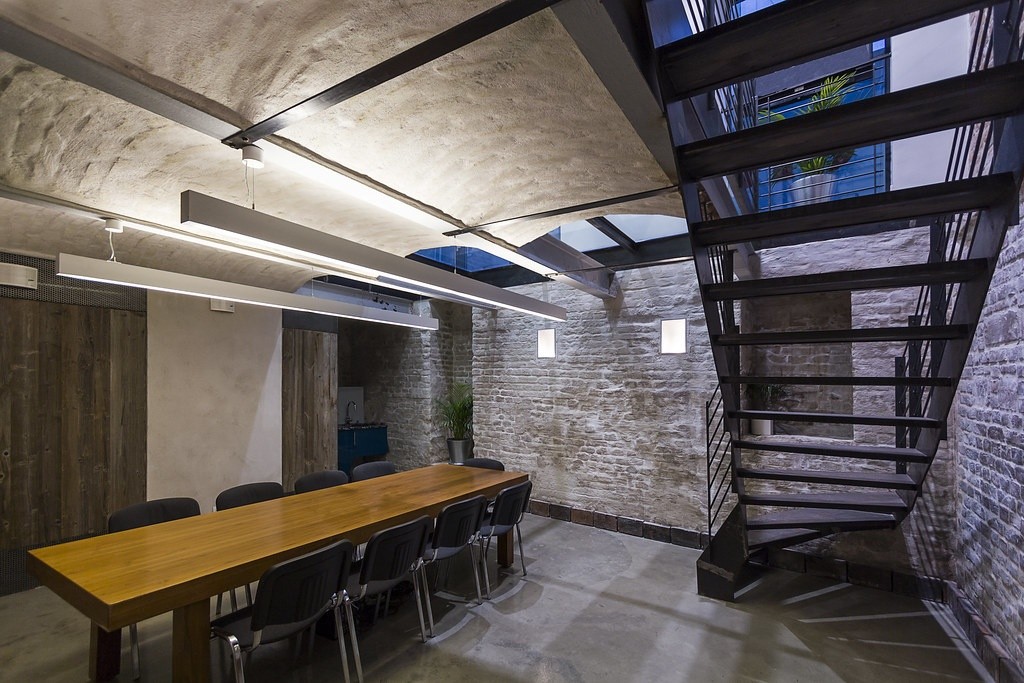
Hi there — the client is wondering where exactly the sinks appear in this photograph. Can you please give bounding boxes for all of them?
[338,427,350,430]
[350,426,377,430]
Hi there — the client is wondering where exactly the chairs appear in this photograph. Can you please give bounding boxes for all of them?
[209,539,356,682]
[309,514,427,683]
[444,480,532,598]
[108,497,201,680]
[352,460,396,483]
[383,494,488,637]
[463,458,505,560]
[214,481,285,617]
[295,470,349,495]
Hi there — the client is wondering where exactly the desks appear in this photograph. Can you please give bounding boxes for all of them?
[26,463,529,683]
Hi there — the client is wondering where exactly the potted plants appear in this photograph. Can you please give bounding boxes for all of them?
[744,366,805,435]
[758,67,873,205]
[434,382,473,463]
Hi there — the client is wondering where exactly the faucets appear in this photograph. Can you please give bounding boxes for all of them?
[344,400,357,424]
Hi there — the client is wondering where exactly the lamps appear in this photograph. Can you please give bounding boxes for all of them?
[54,218,437,331]
[179,143,566,323]
